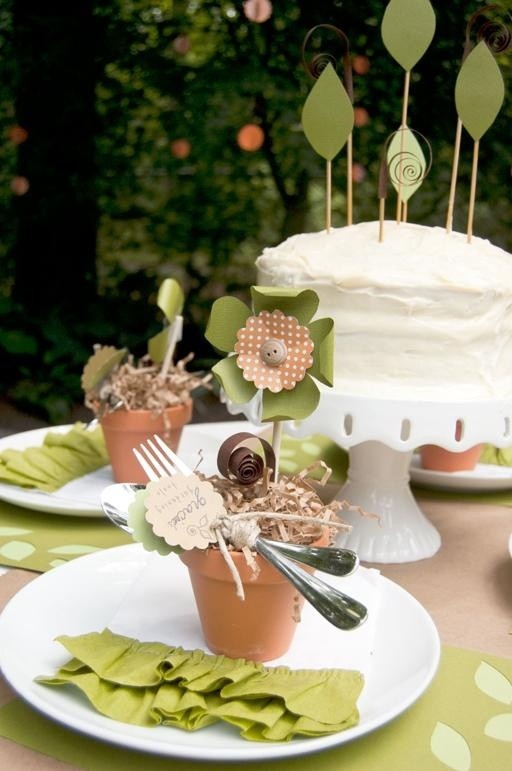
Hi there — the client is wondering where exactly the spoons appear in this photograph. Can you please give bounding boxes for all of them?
[101,483,360,577]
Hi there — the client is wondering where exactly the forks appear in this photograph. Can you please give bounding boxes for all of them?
[132,435,369,632]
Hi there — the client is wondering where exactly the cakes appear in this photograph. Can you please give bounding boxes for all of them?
[254,219,512,401]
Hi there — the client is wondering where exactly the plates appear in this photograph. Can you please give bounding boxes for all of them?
[408,455,512,494]
[1,423,117,518]
[0,541,441,764]
